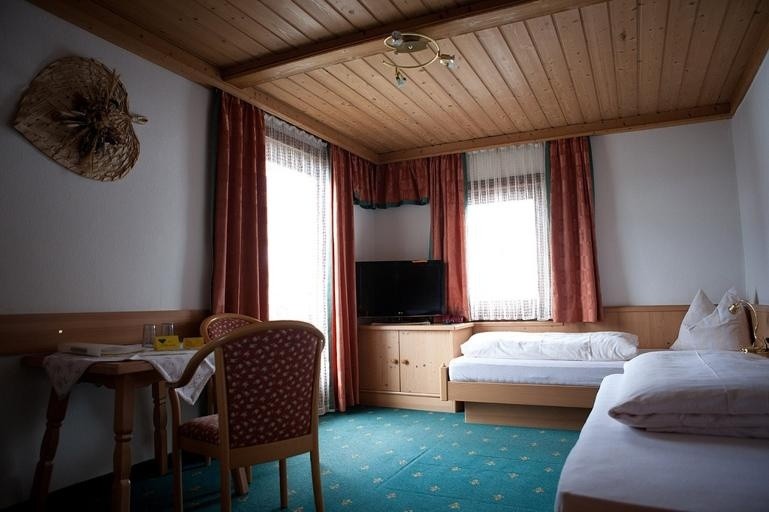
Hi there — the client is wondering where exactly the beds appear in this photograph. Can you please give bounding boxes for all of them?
[441,331,754,428]
[552,375,769,512]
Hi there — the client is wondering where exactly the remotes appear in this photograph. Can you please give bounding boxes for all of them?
[445,320,451,324]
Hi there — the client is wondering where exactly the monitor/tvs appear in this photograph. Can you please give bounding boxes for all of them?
[355,260,443,323]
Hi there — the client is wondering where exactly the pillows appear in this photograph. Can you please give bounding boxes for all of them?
[689,286,752,351]
[670,288,716,350]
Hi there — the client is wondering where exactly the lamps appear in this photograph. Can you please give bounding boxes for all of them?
[728,298,765,352]
[382,30,455,87]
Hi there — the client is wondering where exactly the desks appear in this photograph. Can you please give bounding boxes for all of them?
[30,348,248,512]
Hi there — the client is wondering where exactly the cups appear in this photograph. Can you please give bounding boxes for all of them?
[162,323,174,336]
[142,324,157,348]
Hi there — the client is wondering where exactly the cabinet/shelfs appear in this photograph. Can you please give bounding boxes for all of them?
[355,322,474,413]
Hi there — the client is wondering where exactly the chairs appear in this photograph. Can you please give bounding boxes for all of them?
[163,321,325,512]
[201,313,265,483]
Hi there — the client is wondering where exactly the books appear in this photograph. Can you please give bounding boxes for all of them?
[57,341,143,355]
